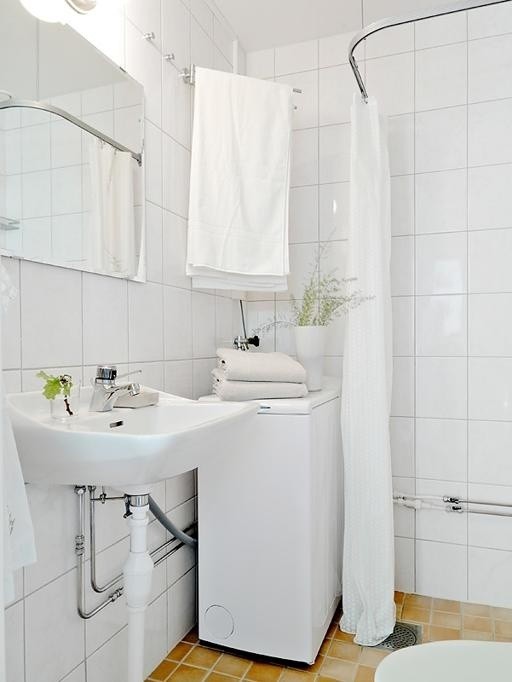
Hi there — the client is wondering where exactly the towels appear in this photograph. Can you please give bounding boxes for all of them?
[209,370,308,401]
[217,347,308,383]
[185,61,297,293]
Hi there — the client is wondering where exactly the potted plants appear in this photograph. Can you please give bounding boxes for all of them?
[251,230,376,393]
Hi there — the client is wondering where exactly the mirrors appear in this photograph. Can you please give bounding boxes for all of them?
[1,0,152,287]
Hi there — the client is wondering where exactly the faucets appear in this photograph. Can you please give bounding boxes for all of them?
[87,364,140,413]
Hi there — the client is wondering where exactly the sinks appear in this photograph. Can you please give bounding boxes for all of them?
[4,384,262,489]
